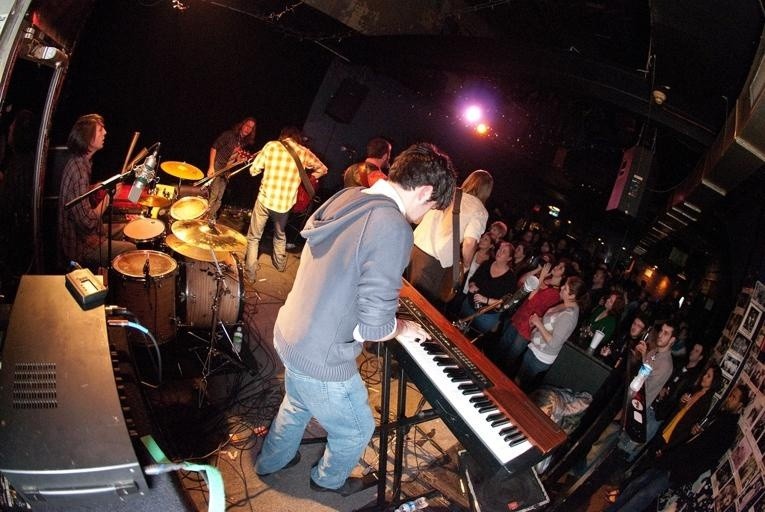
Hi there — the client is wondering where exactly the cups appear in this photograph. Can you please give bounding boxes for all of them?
[699,415,709,428]
[685,392,691,401]
[642,327,657,345]
[589,329,605,349]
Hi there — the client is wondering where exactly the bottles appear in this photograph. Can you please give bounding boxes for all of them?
[629,354,655,393]
[474,290,482,311]
[232,325,247,354]
[394,497,430,512]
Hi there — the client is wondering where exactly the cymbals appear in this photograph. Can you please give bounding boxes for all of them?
[161,161,204,180]
[171,196,208,221]
[166,232,231,262]
[138,196,171,207]
[171,218,248,252]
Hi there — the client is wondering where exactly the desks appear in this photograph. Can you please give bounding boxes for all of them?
[543,333,616,395]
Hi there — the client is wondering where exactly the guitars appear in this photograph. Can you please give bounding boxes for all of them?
[440,259,464,302]
[239,150,318,214]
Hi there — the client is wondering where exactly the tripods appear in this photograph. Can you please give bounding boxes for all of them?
[267,137,310,244]
[183,281,258,407]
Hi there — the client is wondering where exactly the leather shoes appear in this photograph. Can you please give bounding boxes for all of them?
[309,477,364,494]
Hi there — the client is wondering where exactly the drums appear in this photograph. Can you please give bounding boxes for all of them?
[124,218,165,249]
[182,253,244,331]
[113,250,178,343]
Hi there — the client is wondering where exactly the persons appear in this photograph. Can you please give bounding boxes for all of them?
[407,170,493,320]
[207,117,257,223]
[450,207,750,511]
[342,138,392,189]
[0,109,38,287]
[244,126,328,284]
[254,143,458,495]
[58,114,137,270]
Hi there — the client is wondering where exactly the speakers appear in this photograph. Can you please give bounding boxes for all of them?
[457,447,551,512]
[323,78,371,124]
[538,333,615,401]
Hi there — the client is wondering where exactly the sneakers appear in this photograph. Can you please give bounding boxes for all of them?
[255,450,302,477]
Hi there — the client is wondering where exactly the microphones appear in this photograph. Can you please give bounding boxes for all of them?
[498,275,541,312]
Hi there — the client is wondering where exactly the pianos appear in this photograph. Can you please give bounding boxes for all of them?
[382,275,567,484]
